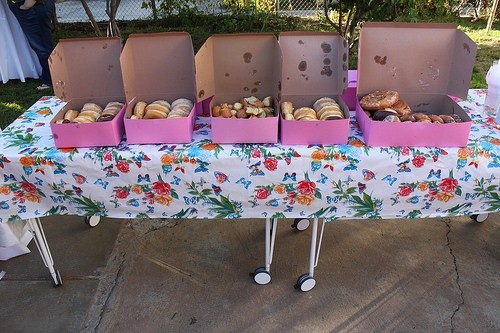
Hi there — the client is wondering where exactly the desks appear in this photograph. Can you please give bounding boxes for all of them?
[0,89,500,292]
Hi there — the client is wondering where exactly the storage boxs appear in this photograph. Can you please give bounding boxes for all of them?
[354,22,478,147]
[47,38,127,148]
[121,31,195,144]
[341,69,360,109]
[280,32,350,144]
[195,33,282,144]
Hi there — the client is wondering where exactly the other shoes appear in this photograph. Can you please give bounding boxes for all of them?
[36,85,50,90]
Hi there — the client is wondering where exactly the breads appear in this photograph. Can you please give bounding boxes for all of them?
[55,101,125,123]
[359,90,463,124]
[212,95,274,119]
[280,97,344,120]
[129,98,193,119]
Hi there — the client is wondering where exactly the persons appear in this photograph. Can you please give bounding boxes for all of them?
[7,0,56,90]
